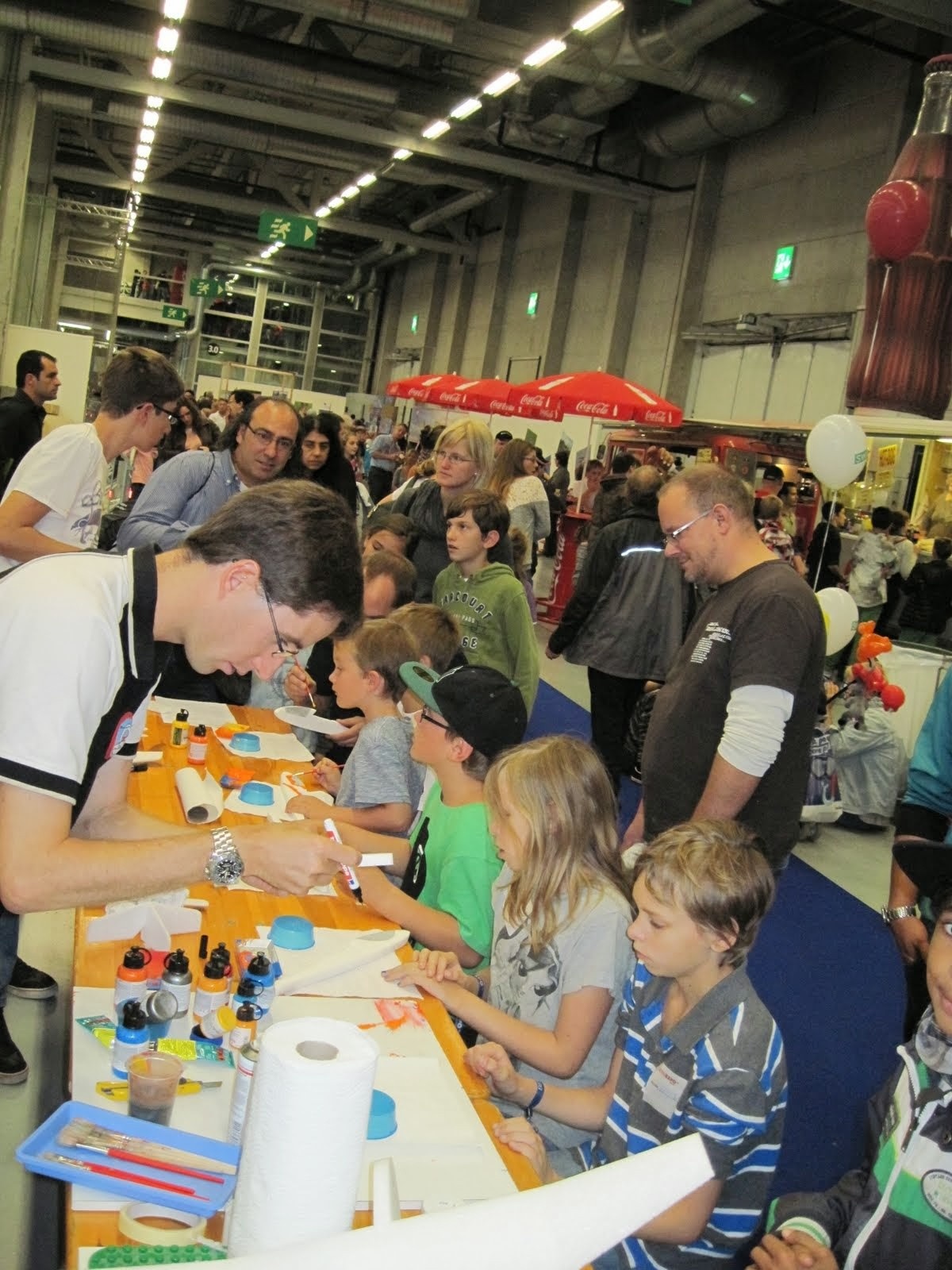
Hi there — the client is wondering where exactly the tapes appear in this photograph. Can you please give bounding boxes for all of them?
[118,1201,207,1247]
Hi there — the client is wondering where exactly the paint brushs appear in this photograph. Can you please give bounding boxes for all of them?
[294,655,316,707]
[37,1150,210,1200]
[290,765,345,777]
[57,1118,237,1183]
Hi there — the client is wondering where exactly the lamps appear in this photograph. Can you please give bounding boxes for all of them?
[261,0,626,259]
[117,0,187,247]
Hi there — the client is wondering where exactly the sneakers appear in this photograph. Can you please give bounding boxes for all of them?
[3,954,58,999]
[0,1011,29,1084]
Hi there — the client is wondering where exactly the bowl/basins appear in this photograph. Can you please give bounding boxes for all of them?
[266,916,316,950]
[238,783,275,805]
[117,997,172,1041]
[229,734,261,752]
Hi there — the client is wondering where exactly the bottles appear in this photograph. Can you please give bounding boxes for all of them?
[113,1000,151,1079]
[243,951,277,1014]
[229,1000,264,1048]
[367,1088,400,1139]
[134,991,178,1023]
[194,1006,237,1039]
[188,724,208,765]
[113,945,151,1012]
[228,1032,264,1146]
[193,942,234,1020]
[169,708,192,748]
[231,976,264,1009]
[159,948,192,1019]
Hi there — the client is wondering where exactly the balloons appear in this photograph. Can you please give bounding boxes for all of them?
[865,180,931,266]
[816,587,859,657]
[805,414,868,490]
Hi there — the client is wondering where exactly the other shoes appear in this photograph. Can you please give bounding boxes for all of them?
[536,549,544,555]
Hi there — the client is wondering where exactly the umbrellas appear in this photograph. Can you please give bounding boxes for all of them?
[422,377,564,423]
[386,373,510,416]
[505,370,682,428]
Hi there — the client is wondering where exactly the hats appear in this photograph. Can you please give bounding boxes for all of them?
[398,661,527,762]
[763,464,784,480]
[890,826,952,897]
[495,430,512,441]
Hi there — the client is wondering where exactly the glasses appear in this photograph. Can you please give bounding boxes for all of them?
[432,449,477,465]
[522,455,536,463]
[136,404,181,425]
[231,558,300,659]
[417,704,454,735]
[246,422,296,452]
[662,509,714,548]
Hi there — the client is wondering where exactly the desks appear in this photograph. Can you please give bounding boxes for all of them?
[67,701,592,1270]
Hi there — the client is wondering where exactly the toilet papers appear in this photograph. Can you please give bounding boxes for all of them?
[227,1014,380,1257]
[175,765,224,824]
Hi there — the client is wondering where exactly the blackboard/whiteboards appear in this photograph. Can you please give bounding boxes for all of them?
[408,407,468,444]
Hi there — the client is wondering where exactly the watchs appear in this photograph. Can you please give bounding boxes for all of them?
[879,904,915,927]
[203,827,244,888]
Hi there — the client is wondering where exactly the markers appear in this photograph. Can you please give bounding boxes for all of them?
[131,764,147,771]
[324,818,363,905]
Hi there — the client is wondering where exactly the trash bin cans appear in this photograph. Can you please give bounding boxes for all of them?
[875,640,952,800]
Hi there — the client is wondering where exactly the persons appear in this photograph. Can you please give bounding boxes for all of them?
[0,346,952,1270]
[466,816,788,1270]
[618,464,828,879]
[430,484,541,727]
[744,885,952,1270]
[0,481,364,919]
[0,350,62,503]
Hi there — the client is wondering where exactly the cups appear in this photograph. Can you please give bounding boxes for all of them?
[126,1052,184,1128]
[190,1027,223,1046]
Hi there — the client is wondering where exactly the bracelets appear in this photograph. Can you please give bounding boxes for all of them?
[473,973,484,999]
[518,1080,545,1120]
